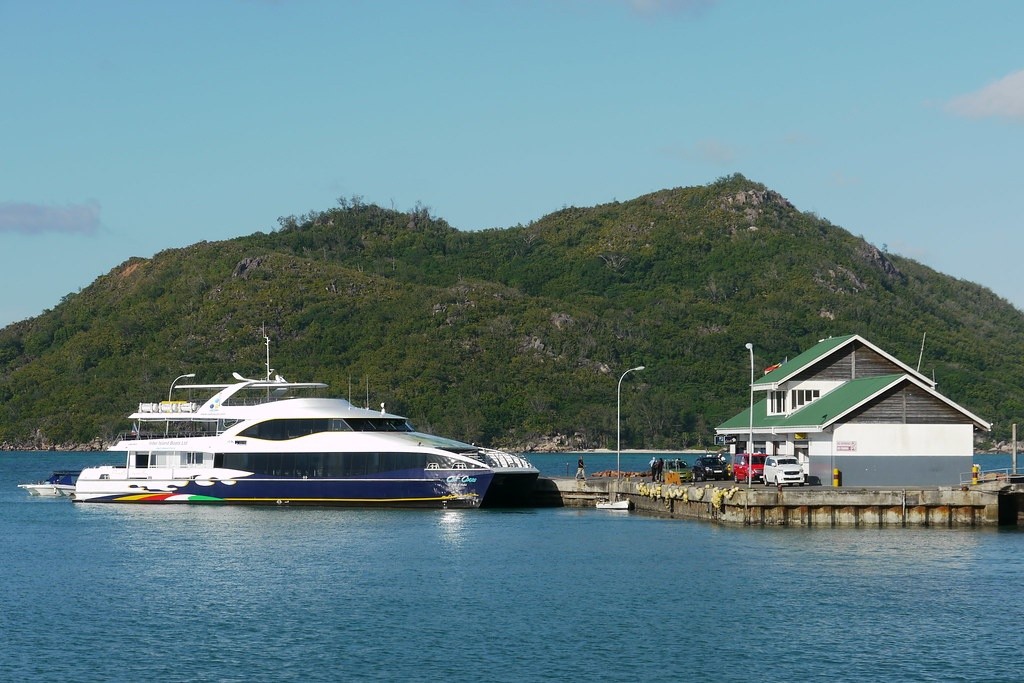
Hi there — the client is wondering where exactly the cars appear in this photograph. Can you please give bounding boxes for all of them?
[661,459,693,483]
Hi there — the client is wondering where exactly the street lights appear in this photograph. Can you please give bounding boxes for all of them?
[744,341,755,489]
[163,372,196,437]
[617,364,646,498]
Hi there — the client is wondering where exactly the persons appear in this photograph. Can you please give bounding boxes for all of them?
[575,456,587,480]
[649,457,664,482]
[719,454,725,461]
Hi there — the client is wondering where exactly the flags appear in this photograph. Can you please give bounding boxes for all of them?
[131,425,136,435]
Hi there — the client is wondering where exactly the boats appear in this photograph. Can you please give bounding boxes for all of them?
[70,333,540,508]
[16,470,82,498]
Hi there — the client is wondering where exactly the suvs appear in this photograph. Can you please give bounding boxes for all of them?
[693,457,729,482]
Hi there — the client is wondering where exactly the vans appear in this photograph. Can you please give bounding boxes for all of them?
[763,455,805,487]
[733,454,768,484]
